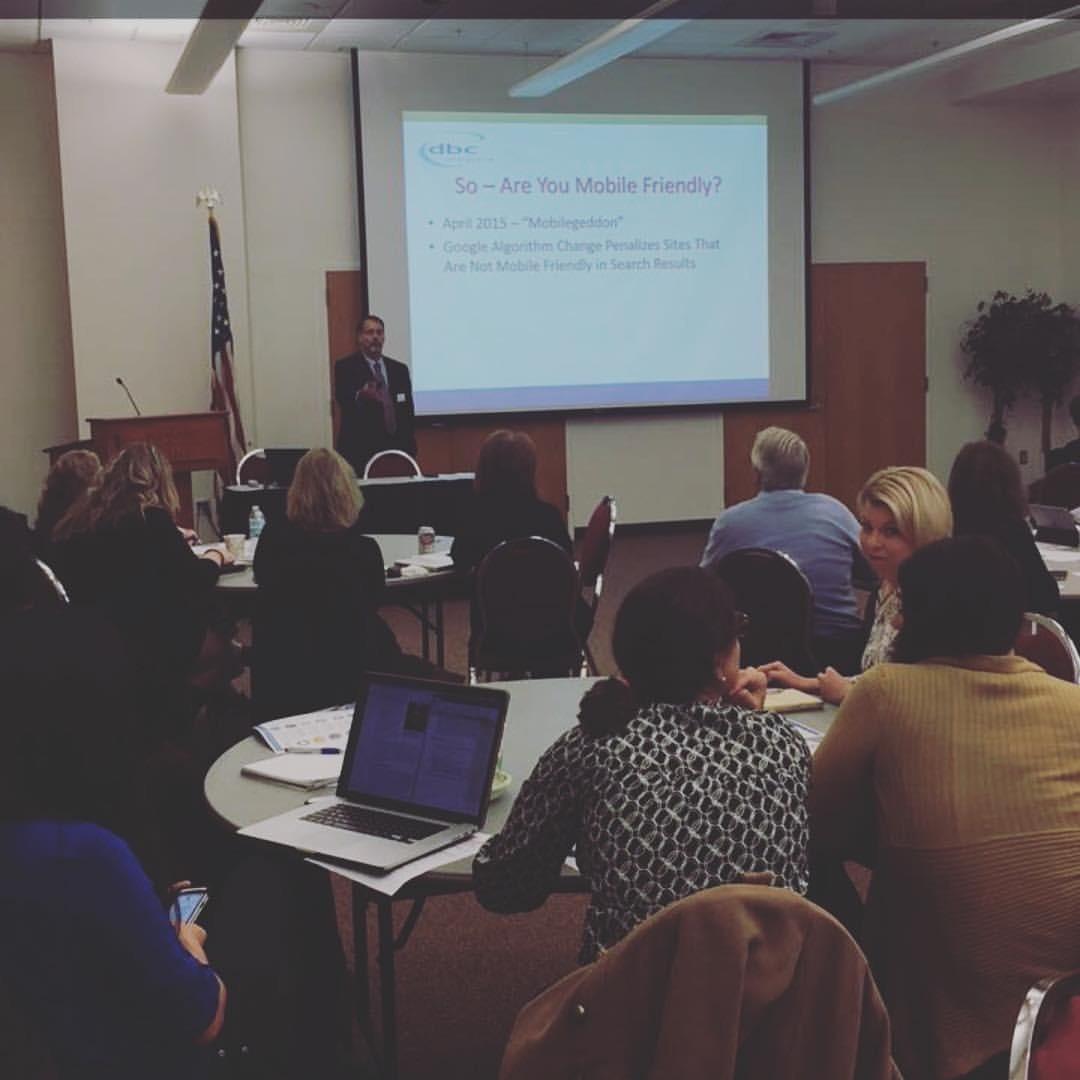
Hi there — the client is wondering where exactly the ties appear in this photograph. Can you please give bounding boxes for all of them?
[373,363,397,437]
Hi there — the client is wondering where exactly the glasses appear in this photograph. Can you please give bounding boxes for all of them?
[727,610,752,642]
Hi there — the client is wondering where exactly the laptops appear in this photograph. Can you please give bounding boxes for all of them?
[236,670,512,872]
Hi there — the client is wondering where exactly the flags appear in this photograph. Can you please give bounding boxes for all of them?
[207,217,249,540]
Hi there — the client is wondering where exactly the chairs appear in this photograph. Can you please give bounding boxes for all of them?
[1009,971,1080,1079]
[24,492,620,677]
[494,880,894,1080]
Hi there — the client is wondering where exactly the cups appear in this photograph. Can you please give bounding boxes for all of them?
[224,534,246,561]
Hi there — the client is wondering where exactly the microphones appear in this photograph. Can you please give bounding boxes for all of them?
[116,376,141,417]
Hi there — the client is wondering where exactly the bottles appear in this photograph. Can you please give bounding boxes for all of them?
[249,505,265,552]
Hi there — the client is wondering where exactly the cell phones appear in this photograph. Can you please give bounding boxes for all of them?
[169,886,212,927]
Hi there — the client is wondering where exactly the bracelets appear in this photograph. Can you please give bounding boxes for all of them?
[203,547,225,571]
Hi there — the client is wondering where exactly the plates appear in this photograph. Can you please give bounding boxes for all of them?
[491,770,512,800]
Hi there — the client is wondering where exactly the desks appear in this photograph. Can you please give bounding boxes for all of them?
[221,470,486,543]
[205,678,843,1080]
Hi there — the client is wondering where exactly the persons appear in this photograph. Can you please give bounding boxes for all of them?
[754,465,957,709]
[331,315,417,479]
[51,442,239,766]
[947,442,1063,624]
[1044,396,1080,472]
[696,424,879,681]
[805,530,1080,1080]
[450,430,590,678]
[253,445,409,720]
[27,449,107,578]
[1,673,230,1079]
[471,566,816,969]
